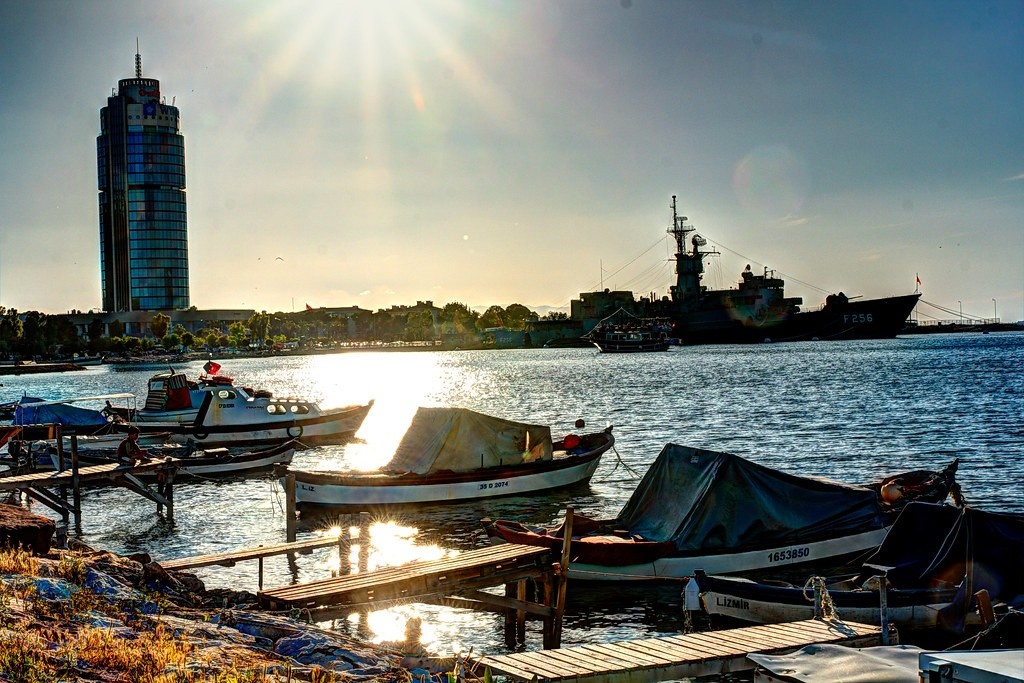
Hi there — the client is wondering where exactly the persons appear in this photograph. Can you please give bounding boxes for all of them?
[117,426,152,465]
[599,321,673,341]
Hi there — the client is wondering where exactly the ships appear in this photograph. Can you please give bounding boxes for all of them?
[481,194,923,349]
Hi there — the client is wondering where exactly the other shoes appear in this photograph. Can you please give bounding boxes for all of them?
[141,457,153,464]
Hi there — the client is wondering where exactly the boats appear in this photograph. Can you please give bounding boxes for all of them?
[678,474,1024,632]
[273,407,616,505]
[45,436,301,478]
[578,306,682,352]
[479,437,969,583]
[0,349,378,470]
[744,588,1024,683]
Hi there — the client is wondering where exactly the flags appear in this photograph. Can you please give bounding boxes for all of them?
[209,360,221,374]
[916,277,921,284]
[306,304,312,310]
